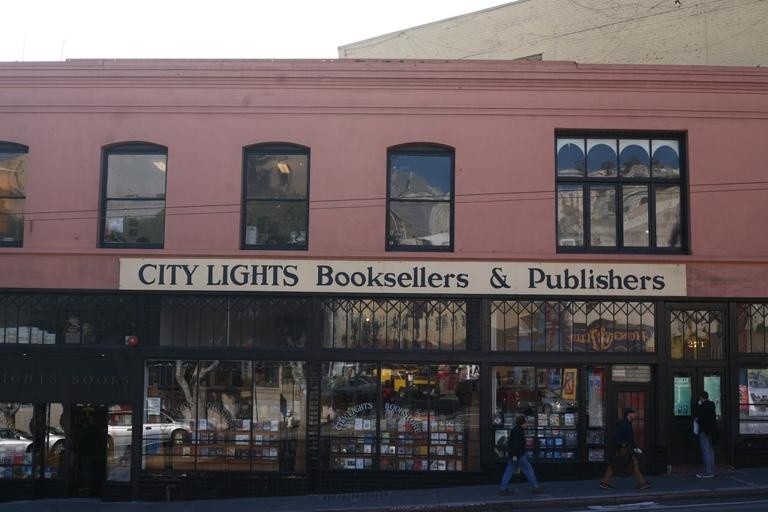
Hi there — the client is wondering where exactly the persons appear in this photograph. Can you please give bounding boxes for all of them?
[495,414,544,496]
[597,407,655,492]
[691,391,720,480]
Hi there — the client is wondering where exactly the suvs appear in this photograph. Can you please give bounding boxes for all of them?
[497,384,536,411]
[107,408,192,451]
[371,365,440,400]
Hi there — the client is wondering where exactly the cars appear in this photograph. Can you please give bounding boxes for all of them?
[532,388,577,414]
[322,374,394,411]
[44,426,67,457]
[454,375,480,405]
[0,427,34,466]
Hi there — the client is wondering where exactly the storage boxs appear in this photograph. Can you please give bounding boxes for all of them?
[144,425,304,471]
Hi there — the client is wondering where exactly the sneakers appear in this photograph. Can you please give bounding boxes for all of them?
[640,481,653,490]
[600,480,616,490]
[696,472,714,479]
